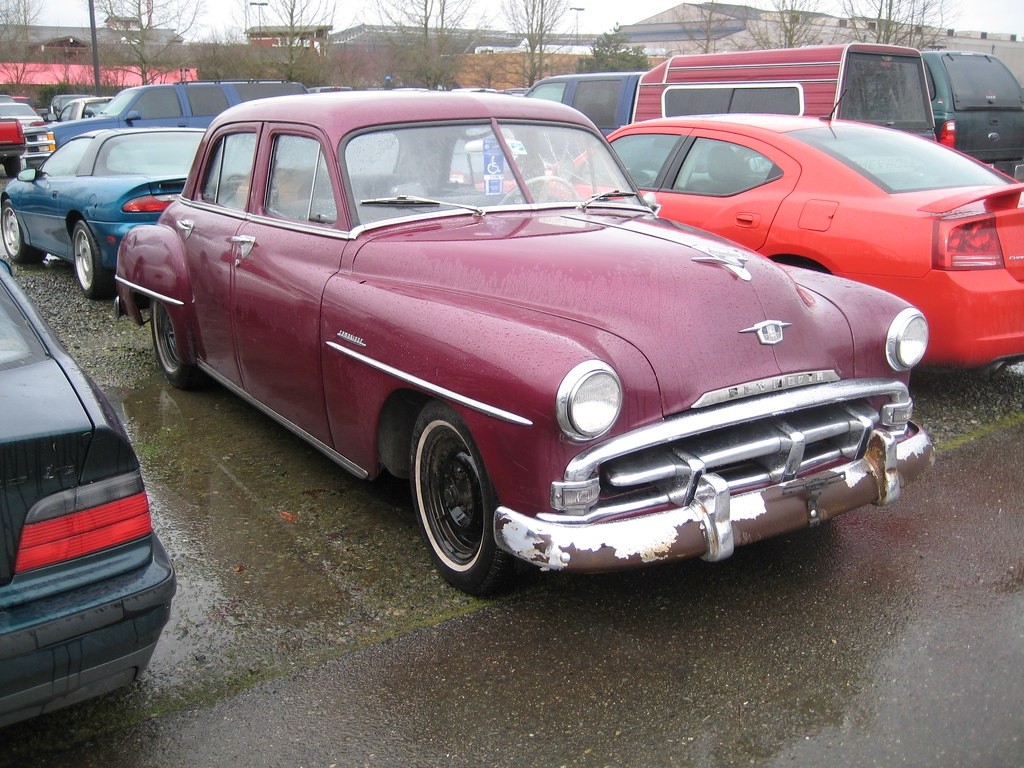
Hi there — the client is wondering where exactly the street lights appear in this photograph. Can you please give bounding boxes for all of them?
[569,7,585,45]
[249,2,269,48]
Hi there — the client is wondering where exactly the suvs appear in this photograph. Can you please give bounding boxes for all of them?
[919,51,1023,189]
[18,78,312,181]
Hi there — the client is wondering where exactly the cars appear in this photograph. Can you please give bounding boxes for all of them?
[0,103,45,136]
[107,88,933,603]
[12,97,37,114]
[0,126,245,298]
[0,264,179,731]
[0,95,16,103]
[51,96,116,125]
[43,94,97,126]
[500,110,1024,378]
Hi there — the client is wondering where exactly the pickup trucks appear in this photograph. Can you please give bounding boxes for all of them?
[0,118,26,179]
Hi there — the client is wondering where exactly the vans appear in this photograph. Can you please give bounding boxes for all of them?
[509,42,939,165]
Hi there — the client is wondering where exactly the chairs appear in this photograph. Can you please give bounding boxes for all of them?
[701,144,751,193]
